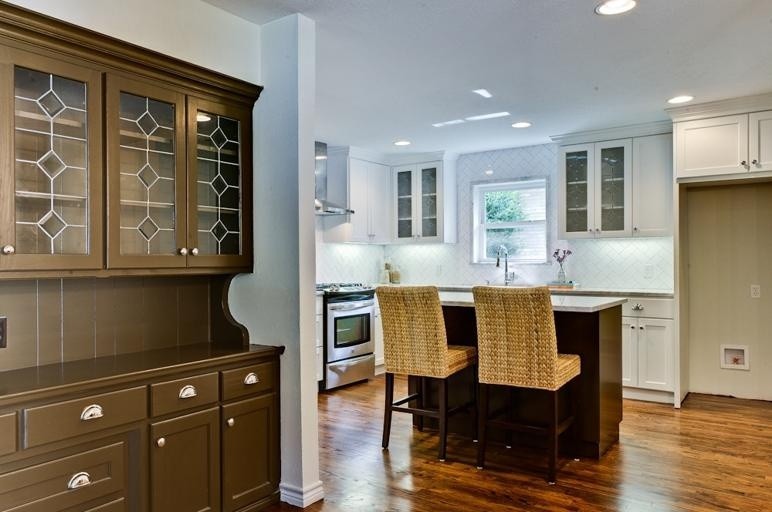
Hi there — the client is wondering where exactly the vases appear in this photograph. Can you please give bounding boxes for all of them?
[558,263,565,281]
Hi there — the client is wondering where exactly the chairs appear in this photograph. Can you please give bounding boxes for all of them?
[375,285,478,462]
[472,285,585,486]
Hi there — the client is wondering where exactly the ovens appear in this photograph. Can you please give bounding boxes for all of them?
[323,294,376,392]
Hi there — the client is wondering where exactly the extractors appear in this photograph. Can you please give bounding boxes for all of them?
[315,141,355,218]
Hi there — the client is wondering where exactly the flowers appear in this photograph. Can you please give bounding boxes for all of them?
[552,248,572,266]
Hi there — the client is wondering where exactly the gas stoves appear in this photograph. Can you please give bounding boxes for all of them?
[315,281,377,297]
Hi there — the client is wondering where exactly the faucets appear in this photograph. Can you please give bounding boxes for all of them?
[495,246,515,286]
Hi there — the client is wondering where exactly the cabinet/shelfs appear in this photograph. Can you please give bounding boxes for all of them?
[0,384,148,512]
[104,72,253,270]
[556,138,632,240]
[148,370,220,512]
[622,296,675,393]
[632,132,674,239]
[316,296,324,382]
[391,150,458,245]
[0,43,104,272]
[673,110,772,179]
[220,359,281,512]
[323,144,391,245]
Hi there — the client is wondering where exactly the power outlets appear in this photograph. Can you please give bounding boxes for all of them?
[750,284,760,297]
